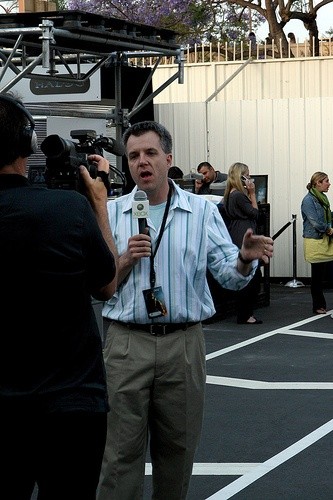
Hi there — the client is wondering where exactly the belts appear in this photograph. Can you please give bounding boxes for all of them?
[116,320,196,337]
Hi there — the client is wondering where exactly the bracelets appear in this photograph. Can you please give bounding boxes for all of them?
[238,251,252,266]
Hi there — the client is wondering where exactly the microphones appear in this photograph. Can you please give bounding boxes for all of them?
[101,136,125,156]
[133,190,149,236]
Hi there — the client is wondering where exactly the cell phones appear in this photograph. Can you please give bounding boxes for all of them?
[240,176,246,186]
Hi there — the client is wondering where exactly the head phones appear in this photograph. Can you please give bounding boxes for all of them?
[0,94,39,158]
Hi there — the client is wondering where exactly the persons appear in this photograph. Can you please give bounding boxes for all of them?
[167,167,184,190]
[194,162,228,198]
[0,90,118,500]
[98,121,275,500]
[301,172,333,314]
[223,163,264,324]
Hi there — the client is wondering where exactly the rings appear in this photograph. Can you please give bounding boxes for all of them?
[264,249,266,254]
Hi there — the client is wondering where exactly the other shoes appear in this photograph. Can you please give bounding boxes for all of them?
[240,316,262,324]
[315,307,326,314]
